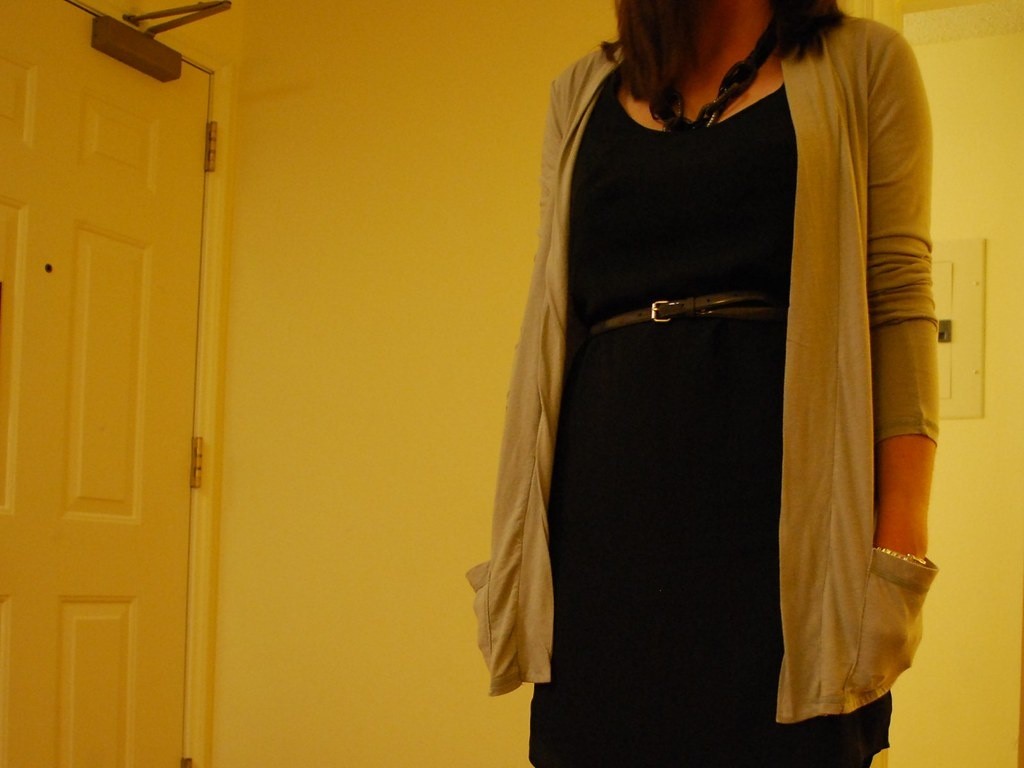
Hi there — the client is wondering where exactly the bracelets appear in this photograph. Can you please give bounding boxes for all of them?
[877,547,926,566]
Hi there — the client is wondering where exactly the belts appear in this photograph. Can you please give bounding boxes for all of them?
[586,289,784,336]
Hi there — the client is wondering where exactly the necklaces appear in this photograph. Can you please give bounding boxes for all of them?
[649,16,779,133]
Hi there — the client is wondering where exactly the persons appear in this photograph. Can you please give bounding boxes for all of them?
[466,0,939,768]
